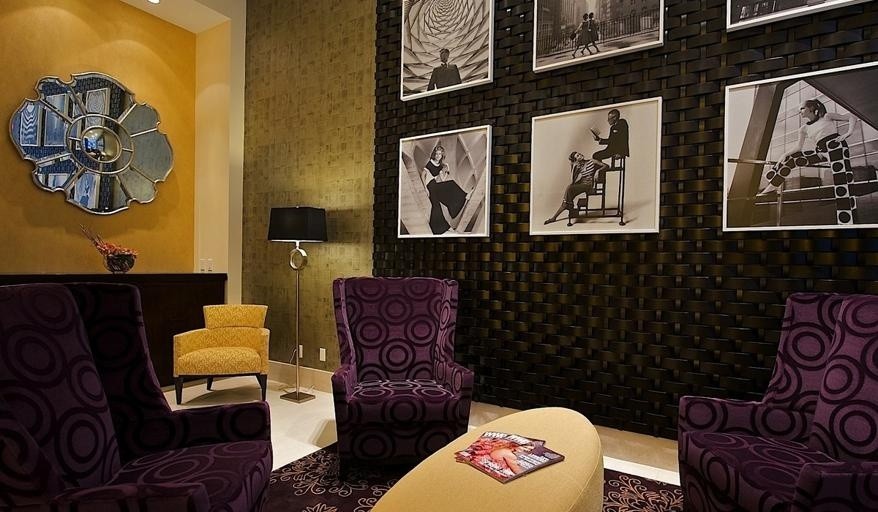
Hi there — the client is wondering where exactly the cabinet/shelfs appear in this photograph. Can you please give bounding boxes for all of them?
[2,273,228,389]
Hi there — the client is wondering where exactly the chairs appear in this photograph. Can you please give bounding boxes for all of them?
[673,291,877,510]
[0,281,275,511]
[68,284,274,459]
[330,274,475,477]
[171,304,271,407]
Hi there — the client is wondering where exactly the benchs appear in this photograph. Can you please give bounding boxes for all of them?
[366,400,606,512]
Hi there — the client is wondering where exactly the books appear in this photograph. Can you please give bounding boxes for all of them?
[455,434,565,484]
[455,429,546,465]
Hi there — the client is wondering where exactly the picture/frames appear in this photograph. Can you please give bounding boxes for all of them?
[73,170,103,211]
[400,1,496,104]
[20,93,44,149]
[720,62,878,232]
[43,94,71,146]
[532,1,665,76]
[725,0,876,36]
[47,172,72,187]
[528,97,663,235]
[85,88,113,132]
[397,125,493,239]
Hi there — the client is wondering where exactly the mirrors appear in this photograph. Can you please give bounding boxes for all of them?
[8,71,174,216]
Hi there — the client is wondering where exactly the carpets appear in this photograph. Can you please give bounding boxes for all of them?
[259,440,688,512]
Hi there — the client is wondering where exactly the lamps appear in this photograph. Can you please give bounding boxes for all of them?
[267,205,328,405]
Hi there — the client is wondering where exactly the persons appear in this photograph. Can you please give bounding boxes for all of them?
[571,14,588,55]
[477,436,534,474]
[582,14,601,56]
[758,99,860,227]
[426,49,462,94]
[589,108,631,176]
[420,145,469,235]
[545,151,609,224]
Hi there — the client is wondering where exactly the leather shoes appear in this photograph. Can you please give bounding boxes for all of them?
[756,191,777,199]
